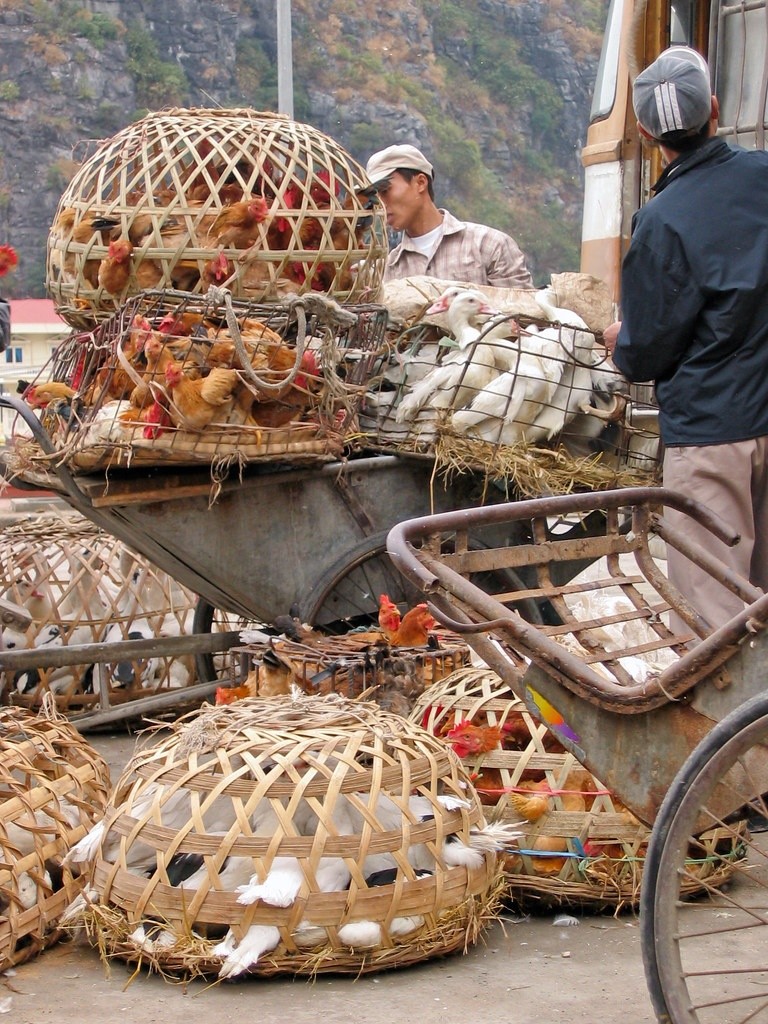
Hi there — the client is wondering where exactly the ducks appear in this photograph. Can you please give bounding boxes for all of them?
[363,287,620,458]
[0,771,528,980]
[2,536,271,699]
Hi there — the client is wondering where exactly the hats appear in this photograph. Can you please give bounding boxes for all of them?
[632,45,712,143]
[344,144,434,203]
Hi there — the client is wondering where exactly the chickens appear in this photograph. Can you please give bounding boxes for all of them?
[217,594,649,891]
[15,148,384,449]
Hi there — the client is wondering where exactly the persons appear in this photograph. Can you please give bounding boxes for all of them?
[362,142,535,291]
[603,45,767,653]
